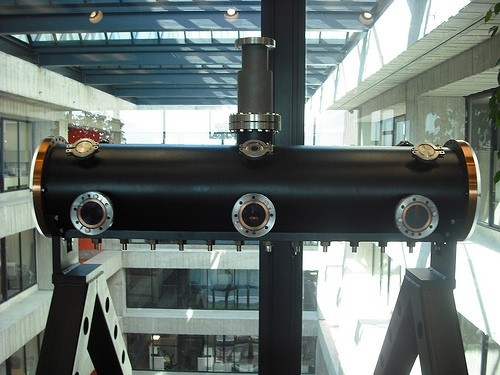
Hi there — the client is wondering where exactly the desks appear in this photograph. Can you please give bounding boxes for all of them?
[4,174,28,191]
